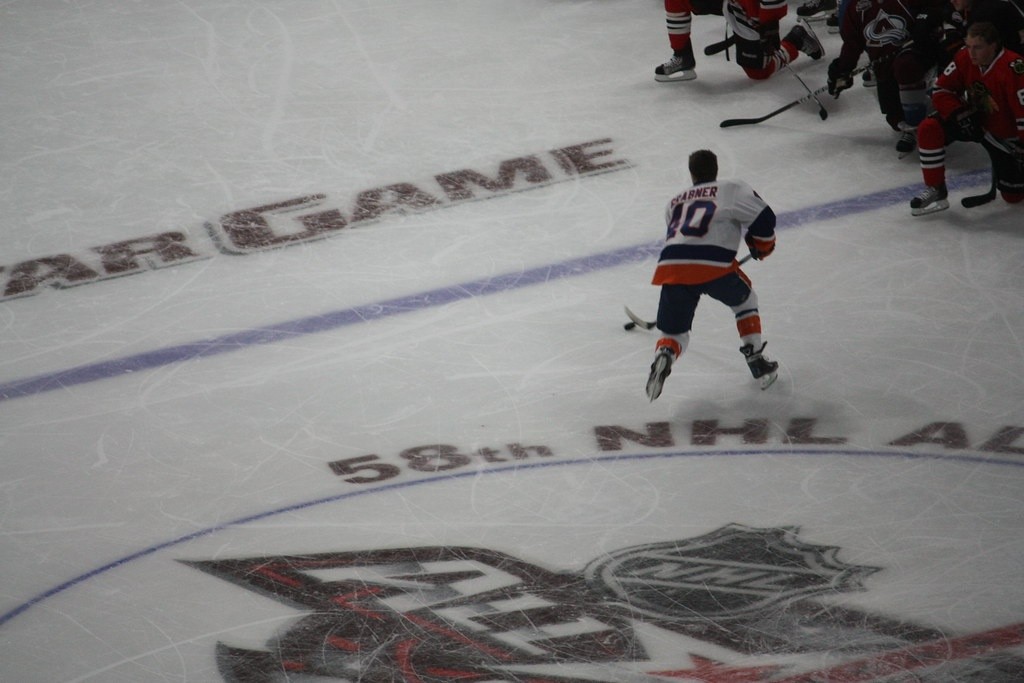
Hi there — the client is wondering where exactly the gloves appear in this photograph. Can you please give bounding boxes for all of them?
[827,59,853,99]
[995,151,1024,194]
[952,106,989,144]
[747,244,774,260]
[911,11,944,50]
[759,20,780,56]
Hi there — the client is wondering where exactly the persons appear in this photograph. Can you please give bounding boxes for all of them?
[654,0,1024,161]
[909,22,1024,216]
[646,150,778,403]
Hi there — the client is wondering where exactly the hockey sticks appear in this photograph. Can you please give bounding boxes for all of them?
[720,49,900,128]
[624,253,752,331]
[732,0,828,121]
[704,34,735,56]
[961,160,998,209]
[725,22,730,61]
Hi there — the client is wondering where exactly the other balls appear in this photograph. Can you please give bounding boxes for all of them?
[624,321,635,331]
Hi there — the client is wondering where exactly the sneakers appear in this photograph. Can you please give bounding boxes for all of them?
[782,19,825,60]
[655,38,697,82]
[909,177,949,216]
[896,121,919,159]
[862,68,877,87]
[796,0,838,22]
[740,341,779,390]
[646,352,672,402]
[826,12,841,35]
